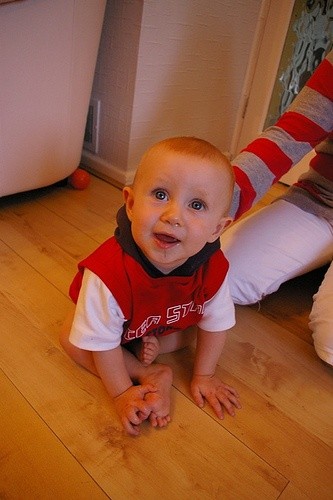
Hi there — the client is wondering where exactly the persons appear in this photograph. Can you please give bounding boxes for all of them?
[220,47,333,365]
[59,136,240,436]
